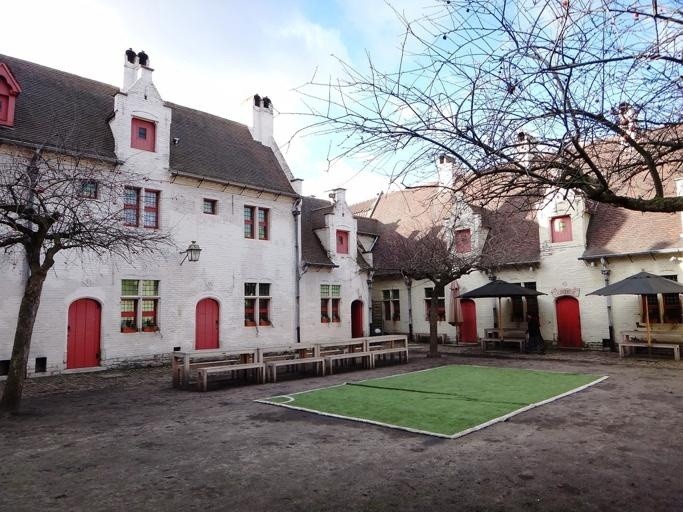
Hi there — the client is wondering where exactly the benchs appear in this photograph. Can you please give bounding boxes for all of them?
[172,328,408,391]
[415,331,447,345]
[618,330,683,360]
[480,327,530,354]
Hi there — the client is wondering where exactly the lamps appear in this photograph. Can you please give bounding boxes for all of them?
[179,240,202,265]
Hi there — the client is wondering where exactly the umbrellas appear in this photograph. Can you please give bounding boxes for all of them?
[452,276,547,337]
[583,268,683,347]
